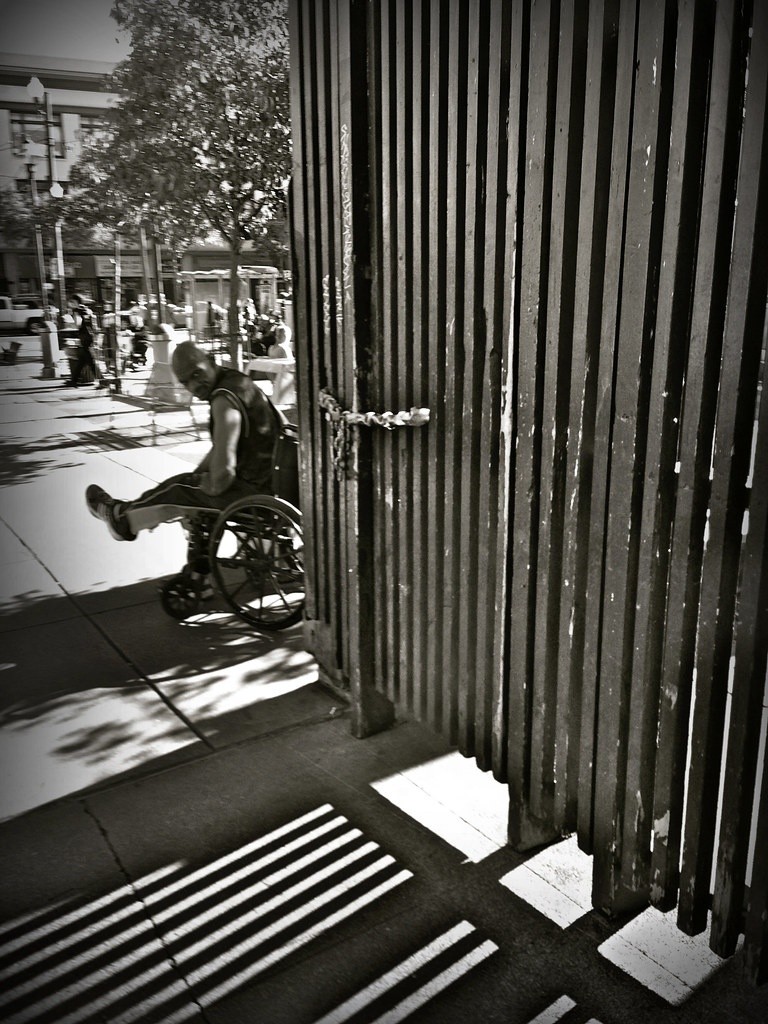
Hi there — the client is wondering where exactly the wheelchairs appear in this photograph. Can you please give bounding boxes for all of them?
[156,419,307,631]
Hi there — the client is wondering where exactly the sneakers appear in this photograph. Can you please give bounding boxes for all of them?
[160,570,215,600]
[86,484,137,541]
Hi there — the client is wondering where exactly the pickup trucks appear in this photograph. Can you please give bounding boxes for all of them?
[0,296,47,337]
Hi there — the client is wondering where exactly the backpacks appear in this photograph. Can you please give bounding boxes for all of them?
[78,314,94,347]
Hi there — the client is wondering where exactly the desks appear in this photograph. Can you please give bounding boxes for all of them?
[245,359,295,407]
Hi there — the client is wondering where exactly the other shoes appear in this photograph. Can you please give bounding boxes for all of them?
[66,381,79,389]
[95,381,109,389]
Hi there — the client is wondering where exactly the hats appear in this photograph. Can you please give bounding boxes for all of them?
[272,310,282,318]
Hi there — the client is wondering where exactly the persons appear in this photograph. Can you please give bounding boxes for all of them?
[43,297,186,329]
[63,292,109,390]
[118,316,149,360]
[236,297,291,356]
[85,341,294,602]
[206,301,216,338]
[100,305,117,374]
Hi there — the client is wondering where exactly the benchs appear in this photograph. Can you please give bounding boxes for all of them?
[109,362,203,446]
